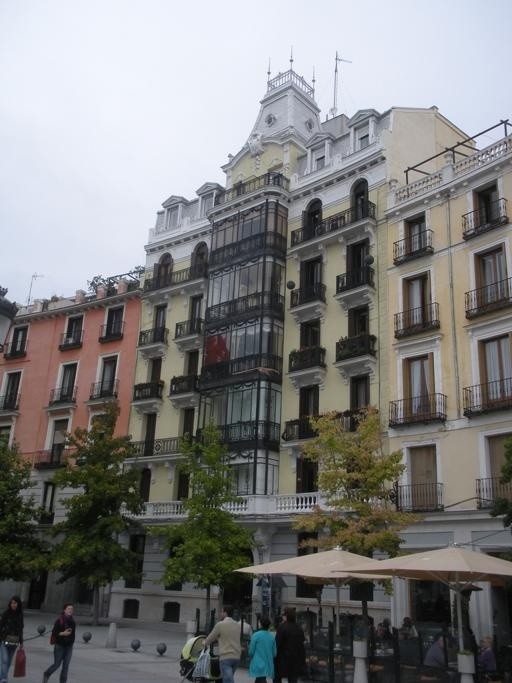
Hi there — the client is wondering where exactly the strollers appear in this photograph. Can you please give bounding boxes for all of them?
[179,636,221,682]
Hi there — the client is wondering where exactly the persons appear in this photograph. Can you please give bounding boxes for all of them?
[42,603,75,683]
[202,606,497,683]
[0,596,24,683]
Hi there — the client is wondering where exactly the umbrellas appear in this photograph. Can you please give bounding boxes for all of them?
[331,543,512,652]
[233,545,398,635]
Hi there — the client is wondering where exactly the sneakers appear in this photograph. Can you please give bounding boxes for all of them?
[43,672,49,683]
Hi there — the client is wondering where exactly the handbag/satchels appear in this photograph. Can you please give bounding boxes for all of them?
[192,644,212,679]
[51,617,63,644]
[13,647,26,677]
[3,634,19,648]
